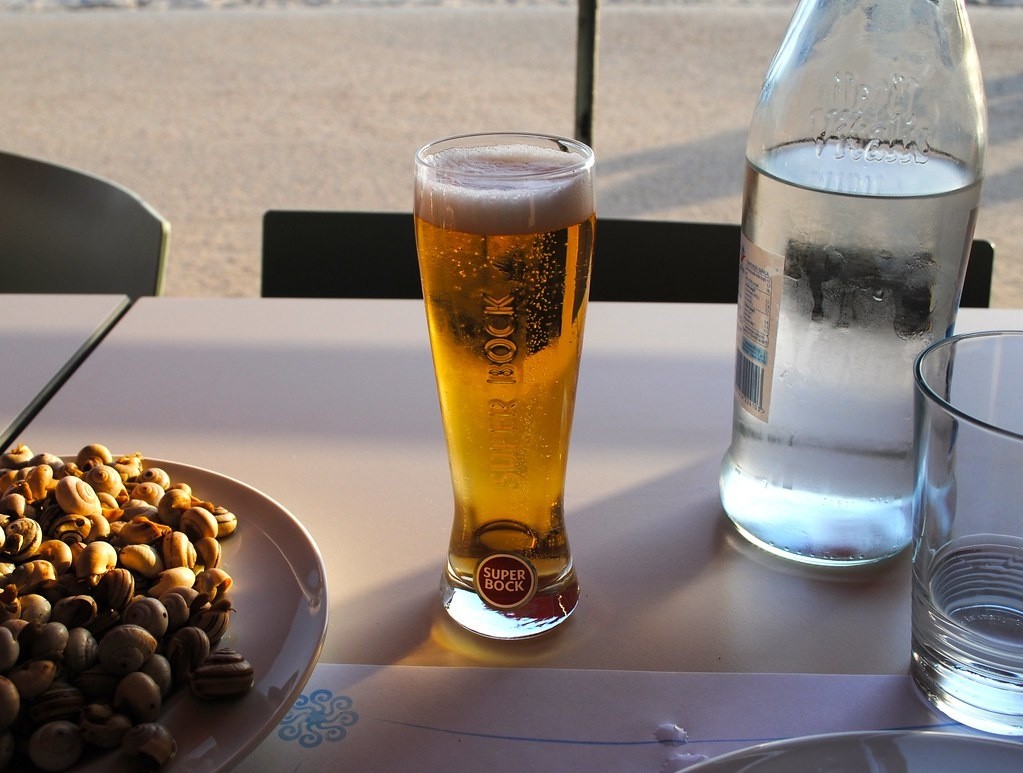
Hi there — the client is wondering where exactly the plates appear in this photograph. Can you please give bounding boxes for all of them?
[676,729,1023,773]
[55,455,330,773]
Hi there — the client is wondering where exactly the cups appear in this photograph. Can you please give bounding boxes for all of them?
[415,132,597,640]
[910,330,1023,738]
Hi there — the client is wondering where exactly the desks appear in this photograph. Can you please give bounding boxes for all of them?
[0,289,1023,773]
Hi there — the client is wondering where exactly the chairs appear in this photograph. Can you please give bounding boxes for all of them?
[0,151,170,296]
[259,209,995,308]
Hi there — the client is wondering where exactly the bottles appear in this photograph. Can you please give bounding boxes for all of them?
[719,0,988,567]
